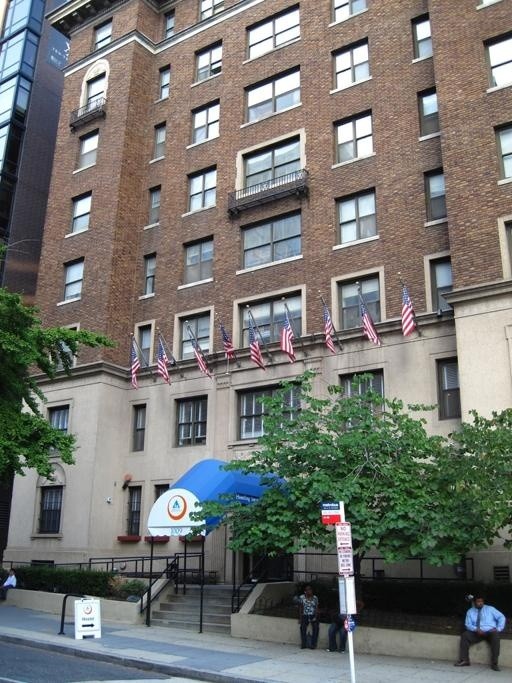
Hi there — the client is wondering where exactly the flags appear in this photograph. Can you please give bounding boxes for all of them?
[186,324,213,381]
[217,322,235,362]
[358,301,382,348]
[398,285,417,338]
[129,339,143,391]
[277,308,299,364]
[154,334,172,385]
[248,313,267,370]
[321,302,337,354]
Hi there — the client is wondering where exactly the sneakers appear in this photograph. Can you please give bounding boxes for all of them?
[326,647,345,654]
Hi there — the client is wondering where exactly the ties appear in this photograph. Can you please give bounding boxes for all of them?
[476,608,481,628]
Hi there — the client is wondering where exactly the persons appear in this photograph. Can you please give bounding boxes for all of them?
[0,567,8,587]
[454,592,508,672]
[296,582,323,651]
[327,597,365,652]
[0,567,17,600]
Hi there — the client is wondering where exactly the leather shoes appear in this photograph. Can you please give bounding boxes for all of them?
[454,659,470,666]
[490,663,500,671]
